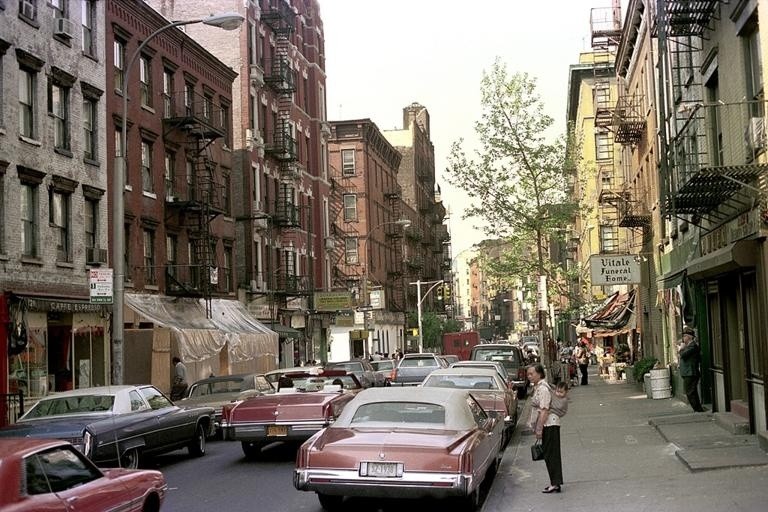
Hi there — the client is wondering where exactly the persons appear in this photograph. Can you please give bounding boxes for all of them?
[353,349,402,360]
[523,341,597,385]
[551,382,568,417]
[171,357,188,401]
[677,328,704,411]
[525,363,563,493]
[295,359,316,366]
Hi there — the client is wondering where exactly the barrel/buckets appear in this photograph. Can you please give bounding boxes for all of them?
[649,367,672,400]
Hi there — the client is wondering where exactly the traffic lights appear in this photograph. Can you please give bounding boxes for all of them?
[437,287,443,301]
[446,287,451,300]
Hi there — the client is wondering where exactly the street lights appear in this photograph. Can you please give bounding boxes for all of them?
[110,12,245,385]
[364,219,411,306]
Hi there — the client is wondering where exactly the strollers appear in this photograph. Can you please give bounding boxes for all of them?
[561,357,579,387]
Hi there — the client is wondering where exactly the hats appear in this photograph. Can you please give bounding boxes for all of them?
[683,329,696,338]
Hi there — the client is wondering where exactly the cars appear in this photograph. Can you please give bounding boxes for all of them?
[293,386,506,510]
[391,343,529,450]
[0,438,167,511]
[1,383,215,469]
[167,359,398,458]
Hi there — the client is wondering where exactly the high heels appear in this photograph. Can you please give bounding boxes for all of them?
[542,485,561,493]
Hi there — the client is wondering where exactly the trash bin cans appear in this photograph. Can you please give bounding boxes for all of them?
[650,369,672,399]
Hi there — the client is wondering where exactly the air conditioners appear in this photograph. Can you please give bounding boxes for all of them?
[54,18,76,39]
[742,117,764,155]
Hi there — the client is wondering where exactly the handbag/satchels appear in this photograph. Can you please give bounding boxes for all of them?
[531,439,544,461]
